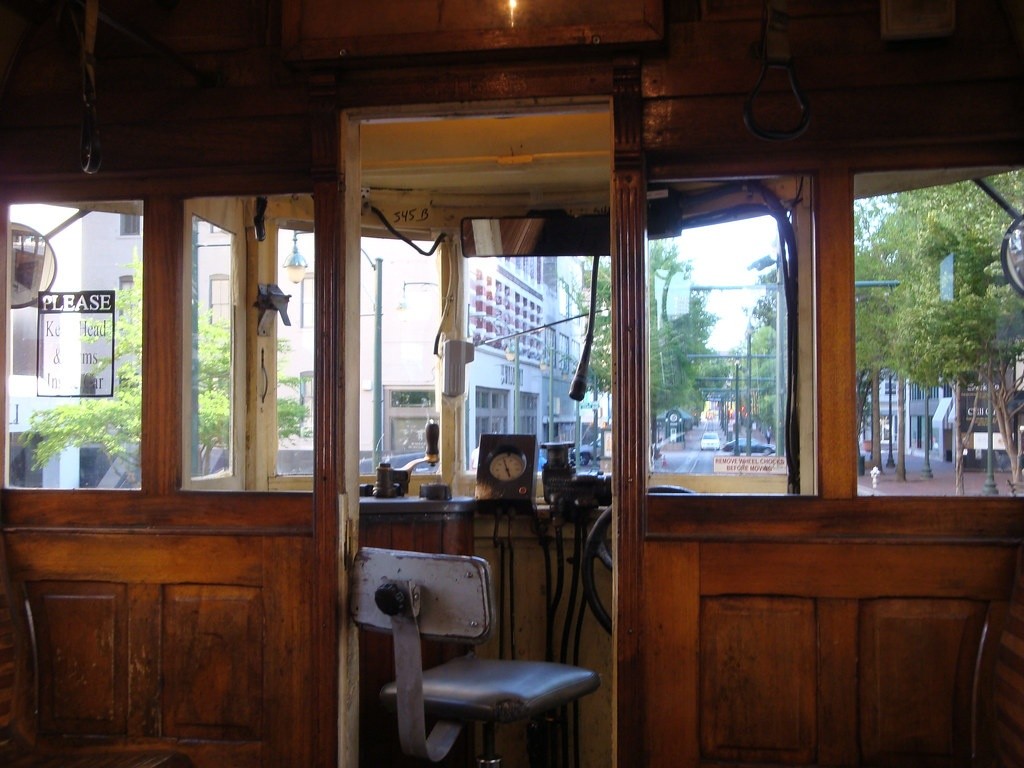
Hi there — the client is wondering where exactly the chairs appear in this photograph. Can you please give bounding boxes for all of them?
[350,545,600,768]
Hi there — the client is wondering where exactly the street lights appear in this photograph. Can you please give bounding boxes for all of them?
[744,320,755,457]
[887,370,895,468]
[734,359,745,456]
[282,227,383,473]
[539,347,554,442]
[504,336,518,435]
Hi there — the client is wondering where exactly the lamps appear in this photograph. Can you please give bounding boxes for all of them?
[283,233,308,284]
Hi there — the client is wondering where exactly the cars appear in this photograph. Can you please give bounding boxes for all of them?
[723,437,775,456]
[700,433,721,451]
[572,436,602,467]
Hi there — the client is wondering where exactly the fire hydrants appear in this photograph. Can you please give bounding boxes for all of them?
[869,467,881,488]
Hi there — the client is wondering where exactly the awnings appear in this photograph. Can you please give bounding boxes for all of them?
[932,397,953,430]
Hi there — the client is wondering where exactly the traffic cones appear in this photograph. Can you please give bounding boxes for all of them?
[663,456,668,467]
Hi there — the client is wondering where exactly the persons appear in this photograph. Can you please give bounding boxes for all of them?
[765,429,772,444]
